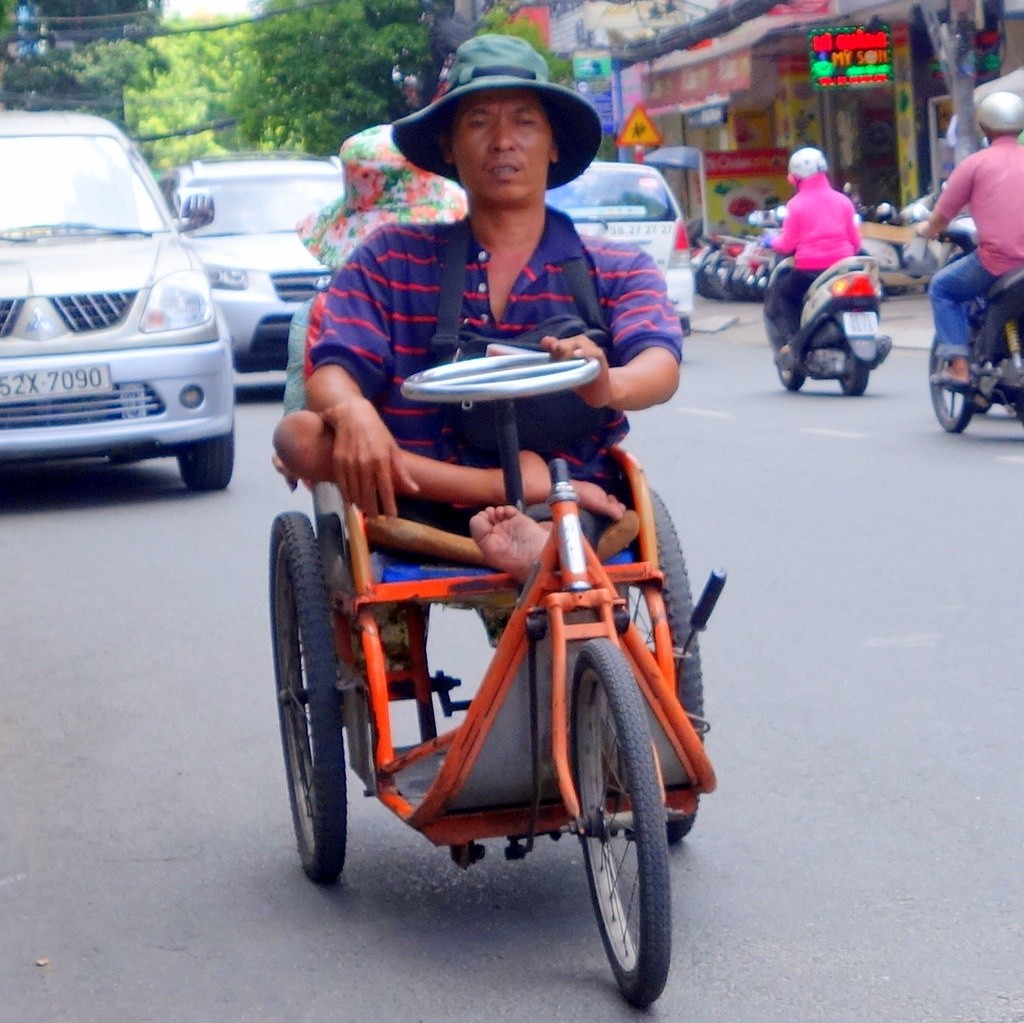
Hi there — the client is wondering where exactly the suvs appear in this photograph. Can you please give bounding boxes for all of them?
[158,156,350,376]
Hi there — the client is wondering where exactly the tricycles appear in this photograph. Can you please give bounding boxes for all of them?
[266,299,727,1007]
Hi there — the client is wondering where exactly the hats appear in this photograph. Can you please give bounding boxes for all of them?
[293,124,468,269]
[392,33,601,190]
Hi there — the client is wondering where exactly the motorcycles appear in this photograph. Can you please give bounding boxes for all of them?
[765,252,894,401]
[906,199,1023,435]
[690,202,940,306]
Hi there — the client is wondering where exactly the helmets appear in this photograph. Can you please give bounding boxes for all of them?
[979,91,1024,134]
[788,147,829,179]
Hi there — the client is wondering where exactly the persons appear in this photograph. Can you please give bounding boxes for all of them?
[760,147,862,341]
[922,91,1024,386]
[305,34,682,585]
[272,123,470,489]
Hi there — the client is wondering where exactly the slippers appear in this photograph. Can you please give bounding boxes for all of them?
[929,370,972,388]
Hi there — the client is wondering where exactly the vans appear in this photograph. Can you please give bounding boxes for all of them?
[1,111,237,492]
[545,161,695,336]
